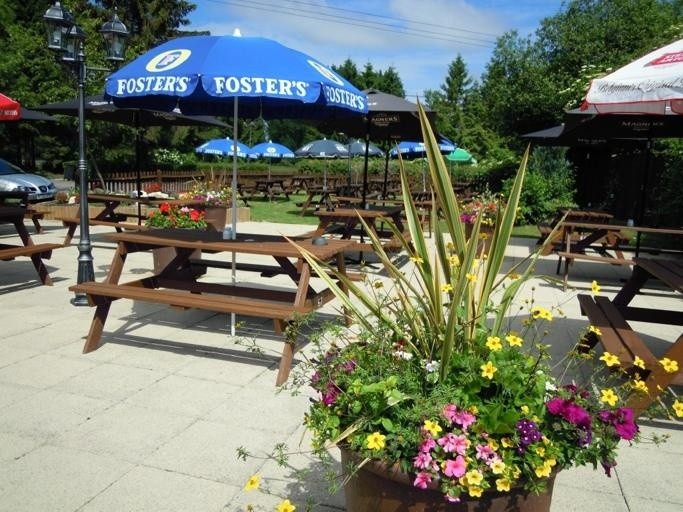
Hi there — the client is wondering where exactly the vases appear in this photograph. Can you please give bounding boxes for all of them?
[335,433,560,511]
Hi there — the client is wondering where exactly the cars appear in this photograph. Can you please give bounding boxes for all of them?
[0,157,57,202]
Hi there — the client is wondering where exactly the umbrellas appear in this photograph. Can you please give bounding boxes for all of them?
[564,38,683,118]
[105,30,370,337]
[196,125,474,188]
[28,94,225,226]
[518,102,683,257]
[0,92,22,122]
[21,107,57,124]
[316,88,443,238]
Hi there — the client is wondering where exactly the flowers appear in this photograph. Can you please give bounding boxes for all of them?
[225,94,683,511]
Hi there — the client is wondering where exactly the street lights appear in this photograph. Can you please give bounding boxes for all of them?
[44,4,129,306]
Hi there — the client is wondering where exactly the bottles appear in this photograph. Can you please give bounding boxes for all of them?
[222,227,231,240]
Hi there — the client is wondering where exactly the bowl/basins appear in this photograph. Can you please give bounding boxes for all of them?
[312,236,327,246]
